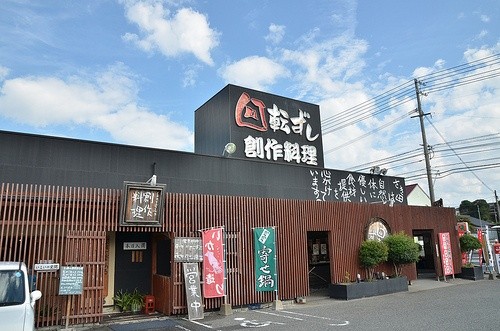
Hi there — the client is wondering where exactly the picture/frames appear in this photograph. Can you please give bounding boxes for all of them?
[120,181,166,228]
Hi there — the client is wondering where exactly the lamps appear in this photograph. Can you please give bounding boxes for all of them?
[222,143,236,155]
[380,168,388,175]
[370,166,379,174]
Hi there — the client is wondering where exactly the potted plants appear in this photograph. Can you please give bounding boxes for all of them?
[330,234,420,299]
[460,235,484,281]
[113,289,144,313]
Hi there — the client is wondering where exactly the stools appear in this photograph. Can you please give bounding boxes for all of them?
[145,295,155,313]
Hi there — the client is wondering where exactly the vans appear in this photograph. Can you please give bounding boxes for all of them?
[0,261,42,331]
